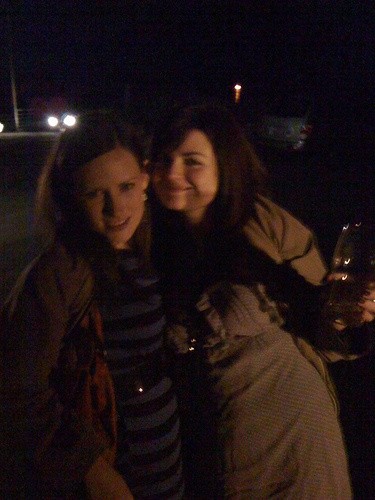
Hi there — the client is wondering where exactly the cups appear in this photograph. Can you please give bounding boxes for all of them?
[321,222,375,333]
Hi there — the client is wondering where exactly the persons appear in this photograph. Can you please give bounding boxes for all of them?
[0,96,375,500]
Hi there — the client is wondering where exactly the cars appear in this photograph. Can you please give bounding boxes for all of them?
[247,117,315,163]
